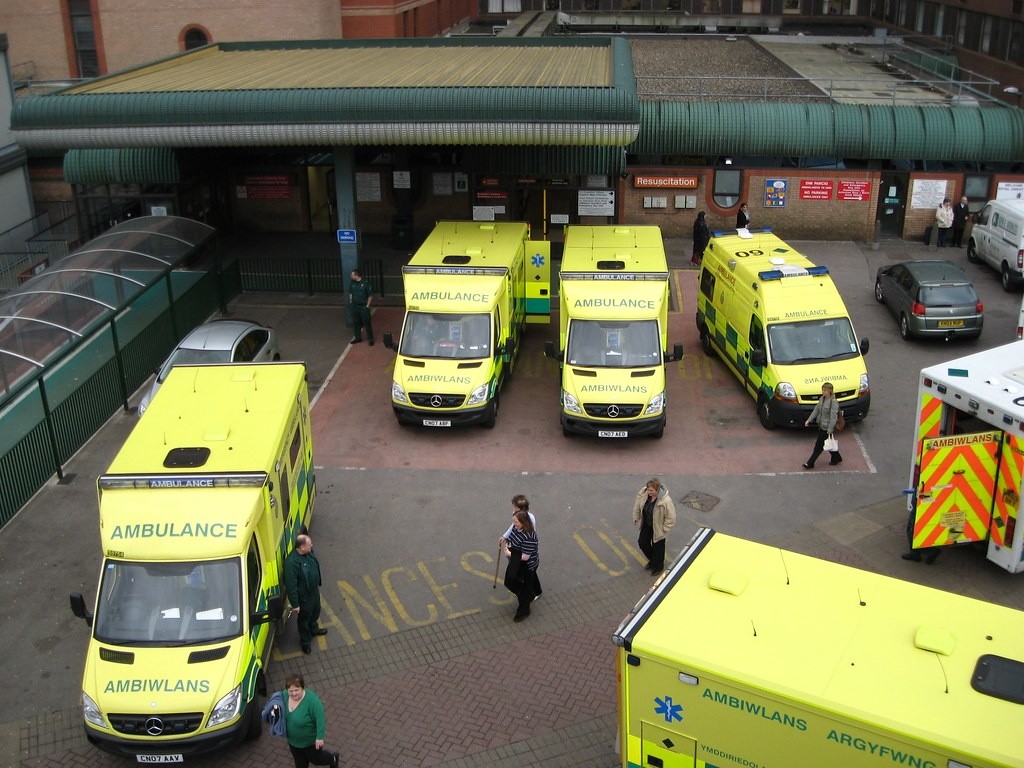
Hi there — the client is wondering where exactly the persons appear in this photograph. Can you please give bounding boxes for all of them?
[283,674,340,768]
[950,195,969,248]
[735,202,751,233]
[499,495,543,624]
[284,534,329,655]
[348,268,375,347]
[413,313,445,356]
[632,478,677,577]
[936,198,954,248]
[802,382,843,470]
[691,211,710,265]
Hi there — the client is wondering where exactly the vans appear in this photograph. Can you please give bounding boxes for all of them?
[967,199,1024,293]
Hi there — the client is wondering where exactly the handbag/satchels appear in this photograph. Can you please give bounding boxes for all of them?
[823,433,839,451]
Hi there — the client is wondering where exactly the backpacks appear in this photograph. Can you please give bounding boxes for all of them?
[834,408,845,433]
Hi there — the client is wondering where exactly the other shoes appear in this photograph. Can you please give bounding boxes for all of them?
[330,752,339,768]
[513,610,531,623]
[957,244,963,249]
[302,643,311,654]
[368,341,374,346]
[644,560,653,571]
[651,567,664,577]
[311,628,327,636]
[948,243,956,248]
[350,338,362,344]
[829,457,842,465]
[941,244,946,248]
[937,241,941,247]
[925,548,941,565]
[901,552,922,562]
[802,464,815,470]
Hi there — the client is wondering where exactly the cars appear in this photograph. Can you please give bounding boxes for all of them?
[874,257,985,342]
[137,318,280,419]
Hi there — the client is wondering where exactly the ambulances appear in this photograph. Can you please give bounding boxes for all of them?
[67,361,318,766]
[545,223,684,439]
[902,340,1024,574]
[694,228,871,432]
[382,220,551,428]
[607,526,1024,768]
